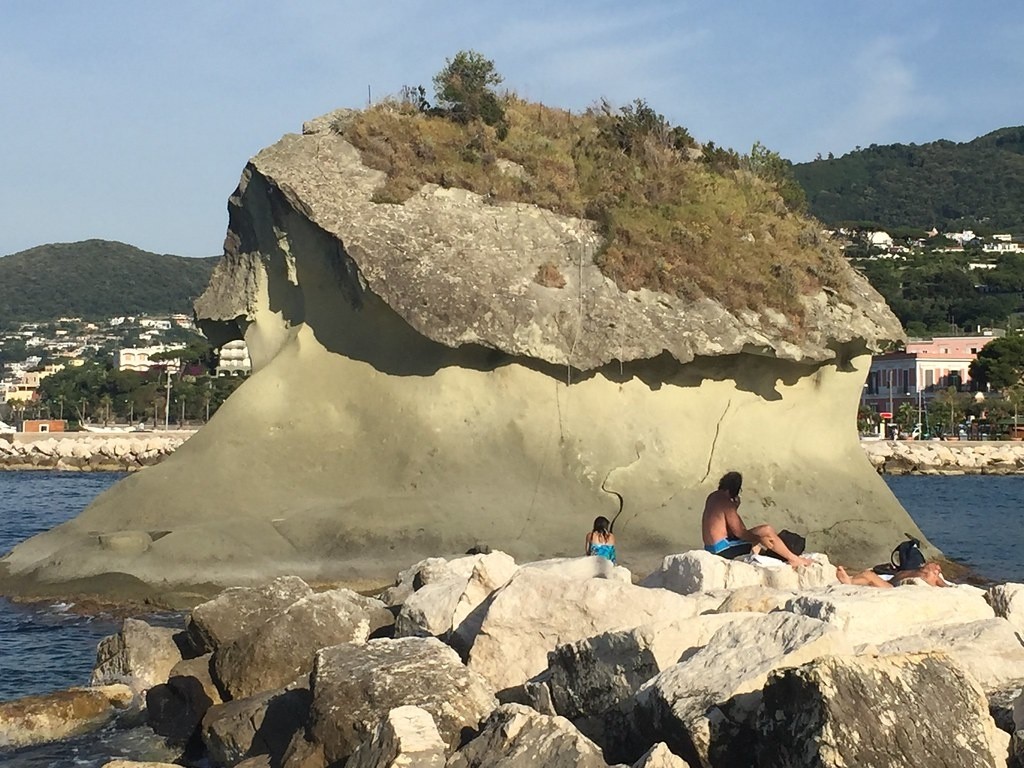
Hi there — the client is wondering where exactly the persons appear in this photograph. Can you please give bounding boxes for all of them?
[585,516,616,564]
[702,472,814,566]
[836,562,951,588]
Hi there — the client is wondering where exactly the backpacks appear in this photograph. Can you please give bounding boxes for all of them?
[890,539,925,570]
